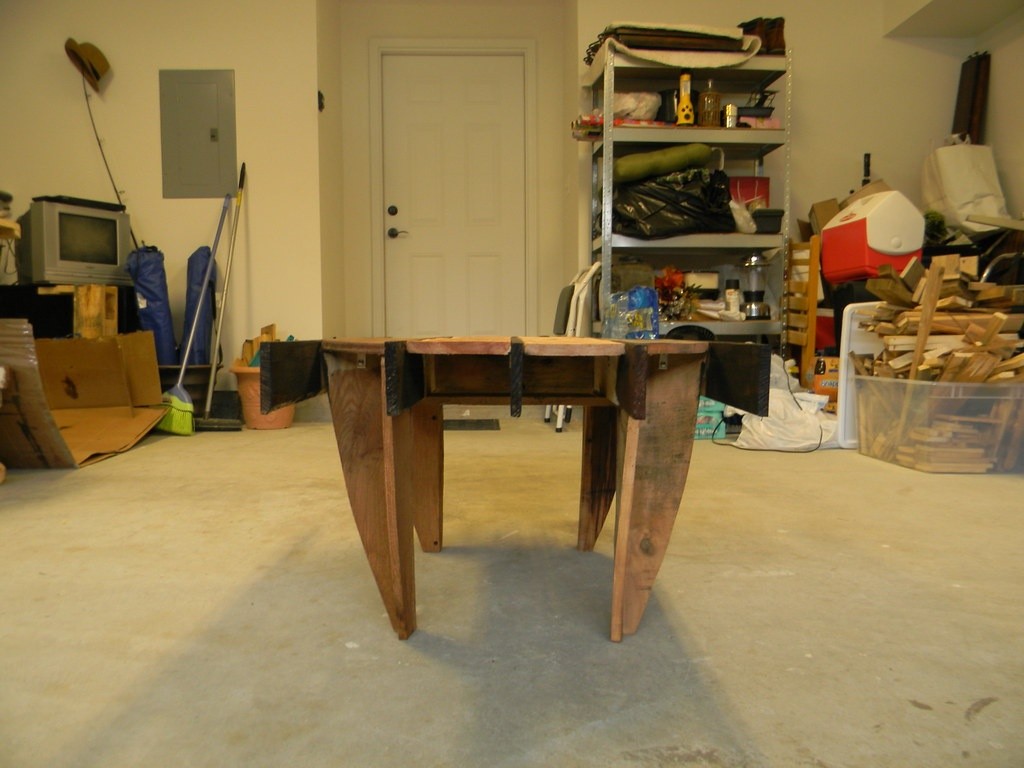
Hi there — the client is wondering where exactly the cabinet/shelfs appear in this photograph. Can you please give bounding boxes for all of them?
[581,34,794,356]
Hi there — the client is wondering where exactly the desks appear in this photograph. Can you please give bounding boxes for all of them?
[257,334,770,649]
[0,282,143,340]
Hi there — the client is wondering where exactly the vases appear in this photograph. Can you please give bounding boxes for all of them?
[158,364,225,419]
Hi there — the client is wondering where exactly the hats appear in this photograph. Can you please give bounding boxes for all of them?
[62,38,110,93]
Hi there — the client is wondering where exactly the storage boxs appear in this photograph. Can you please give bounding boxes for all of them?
[852,372,1024,472]
[750,208,783,234]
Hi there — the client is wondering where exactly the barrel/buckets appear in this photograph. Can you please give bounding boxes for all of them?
[229,366,296,430]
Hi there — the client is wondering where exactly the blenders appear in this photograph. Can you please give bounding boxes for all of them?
[739,249,771,319]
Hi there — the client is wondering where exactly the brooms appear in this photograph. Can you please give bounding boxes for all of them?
[157,193,231,437]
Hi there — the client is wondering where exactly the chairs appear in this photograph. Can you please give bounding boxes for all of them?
[544,261,603,433]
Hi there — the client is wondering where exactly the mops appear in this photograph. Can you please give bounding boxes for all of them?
[195,163,245,432]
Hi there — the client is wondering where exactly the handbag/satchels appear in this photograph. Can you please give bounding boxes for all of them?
[923,133,1012,239]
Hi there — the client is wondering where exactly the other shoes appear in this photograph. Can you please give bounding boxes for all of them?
[765,17,787,53]
[738,17,768,54]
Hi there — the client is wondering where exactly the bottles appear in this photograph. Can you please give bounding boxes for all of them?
[698,79,721,127]
[816,358,826,374]
[725,278,740,313]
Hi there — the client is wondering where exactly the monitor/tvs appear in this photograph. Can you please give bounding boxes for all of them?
[15,200,135,286]
